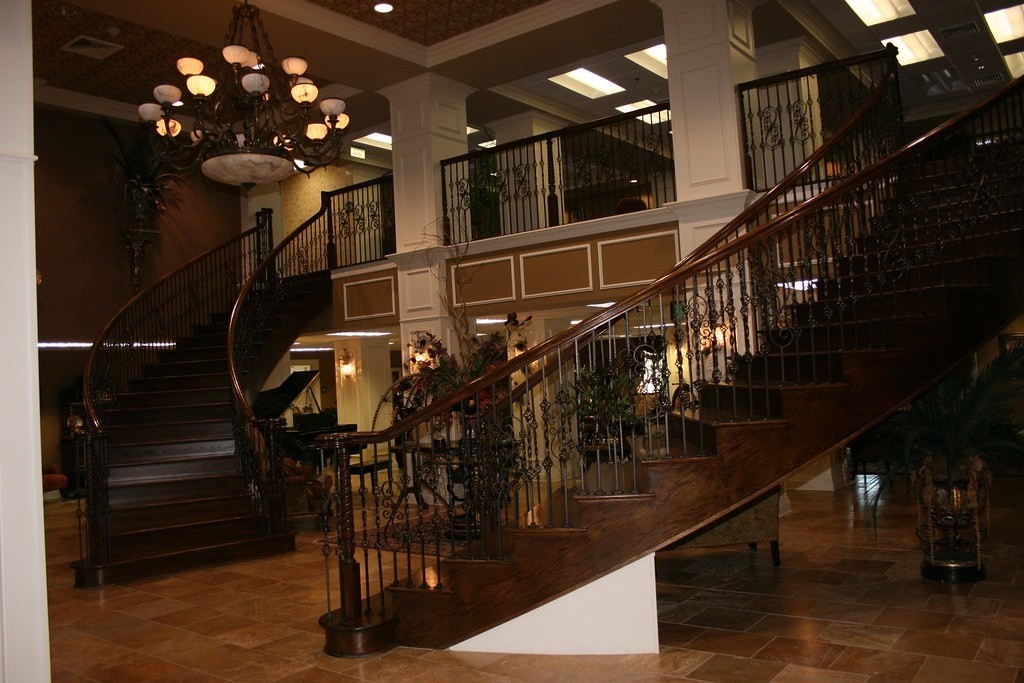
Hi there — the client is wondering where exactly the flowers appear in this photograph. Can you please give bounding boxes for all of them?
[369,215,535,430]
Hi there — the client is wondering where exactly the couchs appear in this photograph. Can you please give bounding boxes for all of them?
[279,456,334,528]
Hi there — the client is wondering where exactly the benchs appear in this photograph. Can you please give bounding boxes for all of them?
[350,459,393,499]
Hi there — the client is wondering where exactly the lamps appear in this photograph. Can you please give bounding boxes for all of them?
[138,0,351,197]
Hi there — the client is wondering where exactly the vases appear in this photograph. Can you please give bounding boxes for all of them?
[460,412,482,445]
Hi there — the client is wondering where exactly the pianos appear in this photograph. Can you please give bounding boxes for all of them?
[250,369,368,493]
